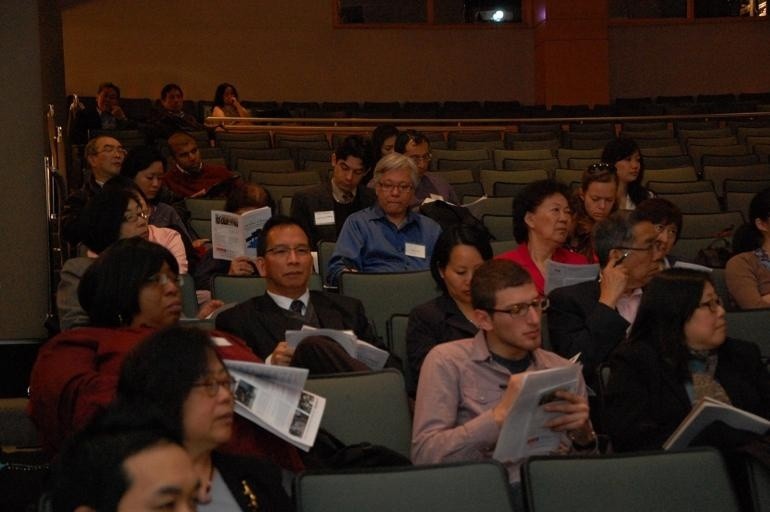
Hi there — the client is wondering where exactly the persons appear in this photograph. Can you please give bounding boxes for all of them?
[80,83,142,130]
[27,239,270,458]
[601,266,770,511]
[54,400,199,510]
[134,84,202,130]
[408,258,598,465]
[206,83,254,126]
[53,124,769,415]
[119,322,296,510]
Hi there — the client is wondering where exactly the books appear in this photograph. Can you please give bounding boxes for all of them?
[493,358,584,463]
[219,356,328,452]
[661,394,769,453]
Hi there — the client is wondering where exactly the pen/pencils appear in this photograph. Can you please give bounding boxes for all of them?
[598,253,628,283]
[498,384,508,388]
[341,258,353,272]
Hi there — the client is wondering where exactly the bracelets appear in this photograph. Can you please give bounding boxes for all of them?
[571,432,596,451]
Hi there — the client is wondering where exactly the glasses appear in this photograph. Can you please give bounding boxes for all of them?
[378,182,415,193]
[697,294,724,313]
[613,241,669,254]
[263,244,313,257]
[120,210,149,223]
[92,147,128,159]
[144,270,186,288]
[192,376,237,398]
[486,294,551,319]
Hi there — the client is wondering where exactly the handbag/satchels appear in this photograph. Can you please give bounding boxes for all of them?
[693,237,733,269]
[300,427,413,469]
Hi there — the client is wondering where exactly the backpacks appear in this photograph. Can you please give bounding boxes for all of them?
[420,200,496,240]
